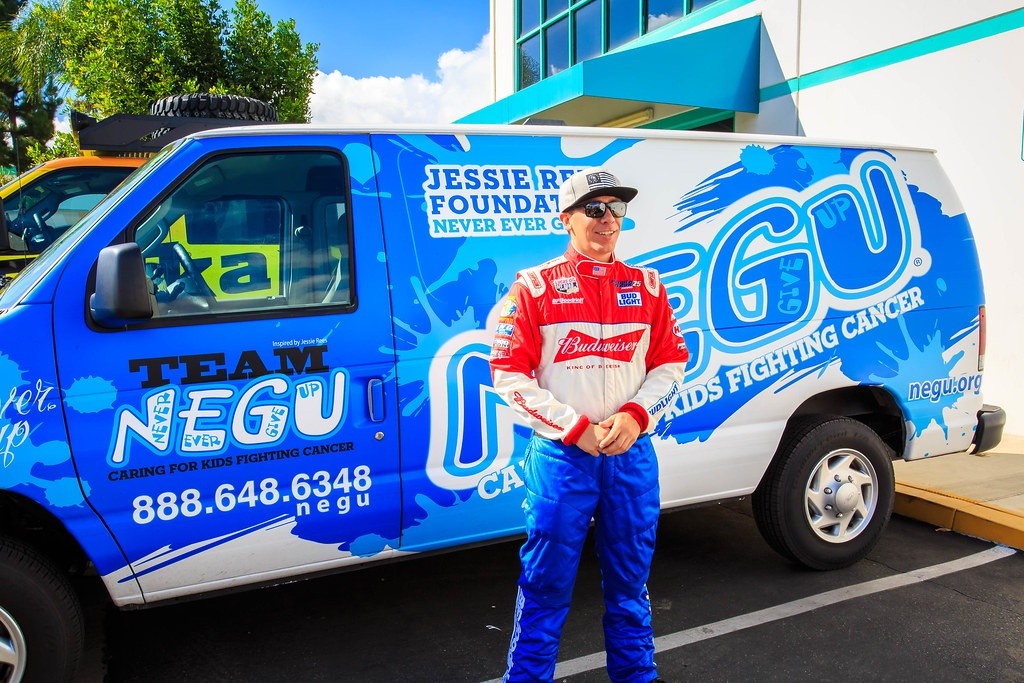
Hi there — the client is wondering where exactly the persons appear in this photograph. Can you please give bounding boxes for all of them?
[487,168,689,683]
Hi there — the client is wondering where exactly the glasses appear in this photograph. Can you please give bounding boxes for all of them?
[572,202,628,218]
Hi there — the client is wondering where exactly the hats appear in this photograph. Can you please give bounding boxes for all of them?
[558,166,638,213]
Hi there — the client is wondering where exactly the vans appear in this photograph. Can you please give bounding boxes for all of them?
[0,127,1006,683]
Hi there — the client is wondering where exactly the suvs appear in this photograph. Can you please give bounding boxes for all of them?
[0,94,275,290]
